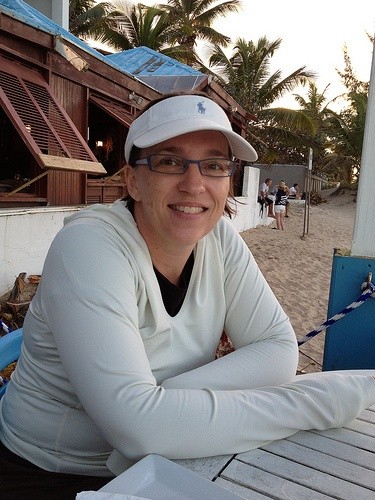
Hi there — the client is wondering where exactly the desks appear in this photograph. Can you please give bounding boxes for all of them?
[170,369,375,500]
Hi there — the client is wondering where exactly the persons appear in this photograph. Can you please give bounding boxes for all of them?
[258,178,300,230]
[0,91,375,500]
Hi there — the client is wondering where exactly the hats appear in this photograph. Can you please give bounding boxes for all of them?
[280,182,285,188]
[124,96,258,164]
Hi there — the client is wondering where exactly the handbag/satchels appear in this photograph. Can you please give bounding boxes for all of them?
[267,194,276,201]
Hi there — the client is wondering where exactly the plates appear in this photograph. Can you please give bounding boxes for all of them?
[98,453,247,500]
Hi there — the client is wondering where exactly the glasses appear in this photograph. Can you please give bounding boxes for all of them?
[134,152,237,177]
